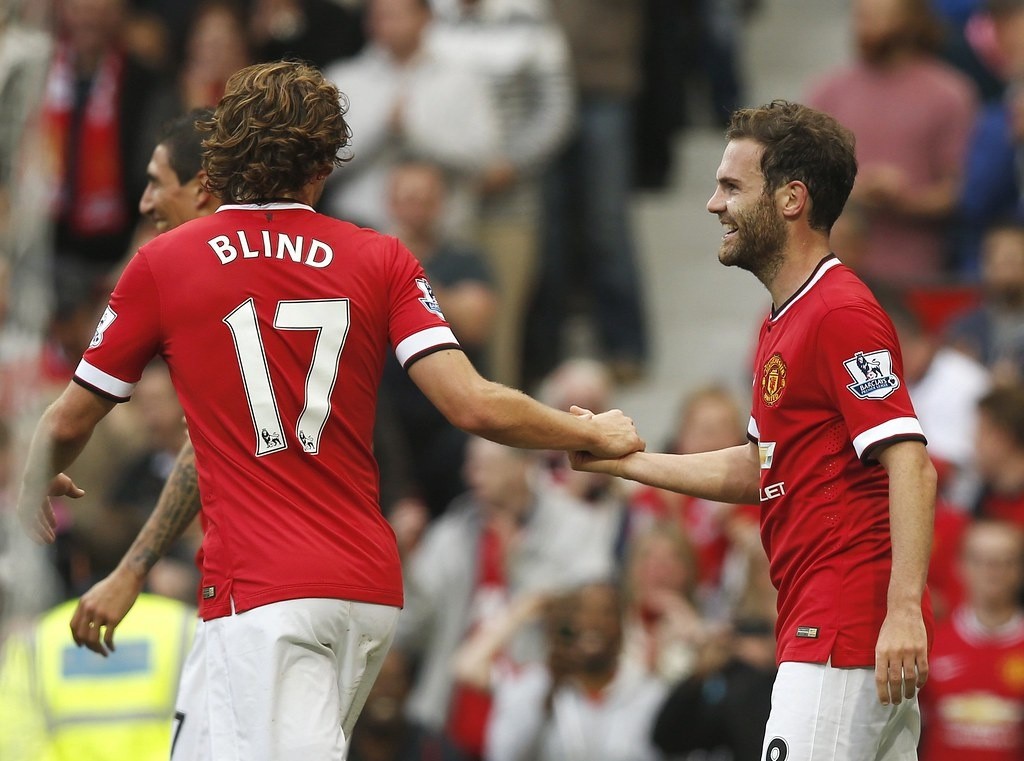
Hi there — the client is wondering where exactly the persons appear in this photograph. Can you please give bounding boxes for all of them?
[69,112,228,657]
[4,1,1023,761]
[571,99,935,759]
[20,62,645,761]
